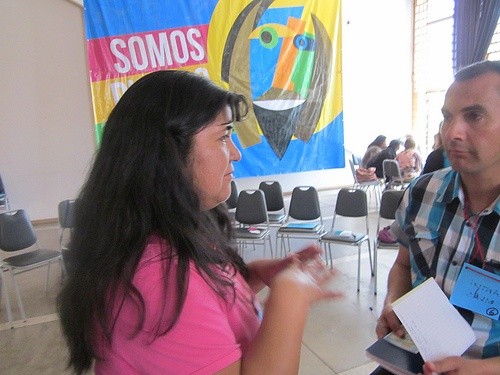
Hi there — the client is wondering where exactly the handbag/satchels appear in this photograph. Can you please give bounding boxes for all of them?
[356,167,377,184]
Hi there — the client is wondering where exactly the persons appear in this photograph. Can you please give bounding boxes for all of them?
[365,59,500,375]
[55,69,345,375]
[367,140,400,191]
[362,135,386,171]
[395,136,422,183]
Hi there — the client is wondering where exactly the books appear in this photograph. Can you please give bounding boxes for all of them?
[365,324,425,374]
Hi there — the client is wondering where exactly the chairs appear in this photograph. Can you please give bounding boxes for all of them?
[372,190,401,296]
[319,188,374,292]
[223,179,325,260]
[0,176,77,329]
[348,152,412,208]
